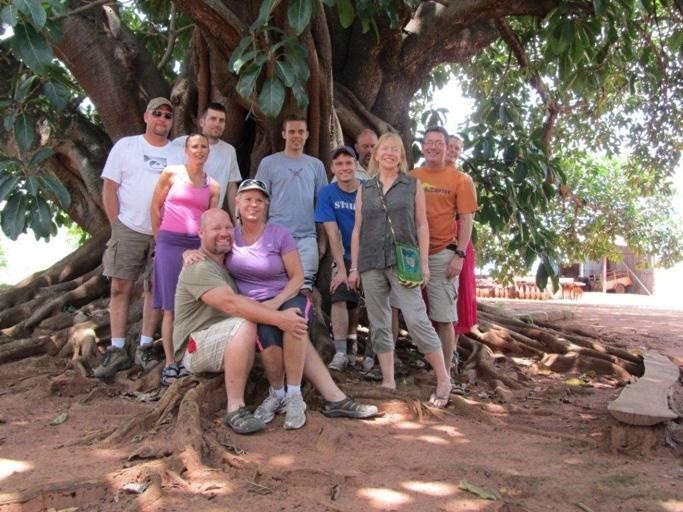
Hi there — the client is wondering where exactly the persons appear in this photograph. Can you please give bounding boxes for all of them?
[180,180,311,430]
[314,124,383,382]
[92,96,181,379]
[155,104,242,358]
[407,126,477,393]
[347,133,453,409]
[412,134,477,369]
[163,207,378,435]
[254,113,327,292]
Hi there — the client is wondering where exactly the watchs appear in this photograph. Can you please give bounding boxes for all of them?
[455,250,466,258]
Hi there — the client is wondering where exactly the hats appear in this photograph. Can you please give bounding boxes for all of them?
[236,178,270,196]
[147,96,175,113]
[330,147,356,160]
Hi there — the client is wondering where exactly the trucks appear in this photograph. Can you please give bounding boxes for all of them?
[575,268,634,293]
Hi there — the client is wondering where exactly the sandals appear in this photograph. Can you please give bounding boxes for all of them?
[160,363,190,386]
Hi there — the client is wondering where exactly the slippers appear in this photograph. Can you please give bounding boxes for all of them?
[430,386,454,409]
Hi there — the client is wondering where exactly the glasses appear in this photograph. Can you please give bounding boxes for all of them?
[151,110,173,119]
[240,179,268,190]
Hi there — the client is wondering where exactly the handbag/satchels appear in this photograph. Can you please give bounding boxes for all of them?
[395,243,424,289]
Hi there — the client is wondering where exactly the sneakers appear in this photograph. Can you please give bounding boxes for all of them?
[451,378,465,395]
[134,343,160,372]
[94,345,132,378]
[328,347,432,380]
[223,388,306,433]
[320,397,379,420]
